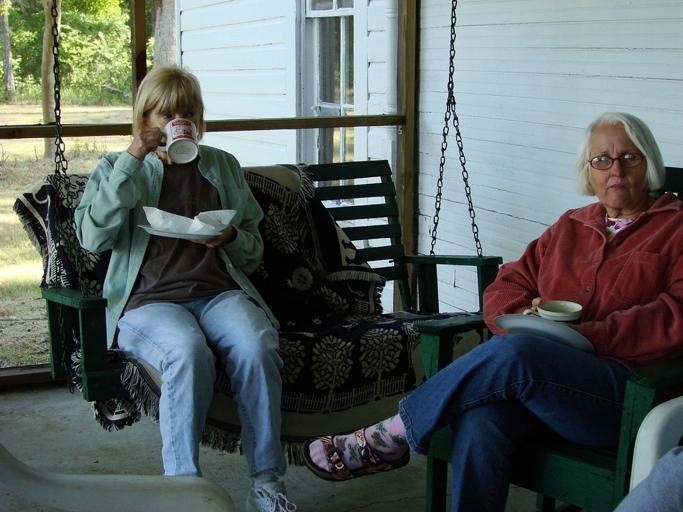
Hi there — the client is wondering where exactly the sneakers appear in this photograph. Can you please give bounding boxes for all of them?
[244,479,297,511]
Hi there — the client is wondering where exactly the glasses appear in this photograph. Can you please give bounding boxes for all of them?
[587,151,644,171]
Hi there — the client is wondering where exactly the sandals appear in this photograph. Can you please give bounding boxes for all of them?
[300,427,410,482]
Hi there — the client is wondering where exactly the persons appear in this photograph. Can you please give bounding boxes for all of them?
[74,63,300,511]
[299,111,683,511]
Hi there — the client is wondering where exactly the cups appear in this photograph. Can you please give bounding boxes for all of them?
[157,118,200,164]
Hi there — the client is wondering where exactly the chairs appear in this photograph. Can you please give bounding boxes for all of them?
[412,168,683,512]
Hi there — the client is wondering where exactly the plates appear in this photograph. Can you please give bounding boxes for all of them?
[492,311,595,359]
[131,224,223,241]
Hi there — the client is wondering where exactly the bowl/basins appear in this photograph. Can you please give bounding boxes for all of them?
[536,300,582,321]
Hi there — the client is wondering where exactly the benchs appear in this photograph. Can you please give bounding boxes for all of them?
[14,159,503,466]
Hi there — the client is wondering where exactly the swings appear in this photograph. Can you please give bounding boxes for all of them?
[14,0,504,464]
